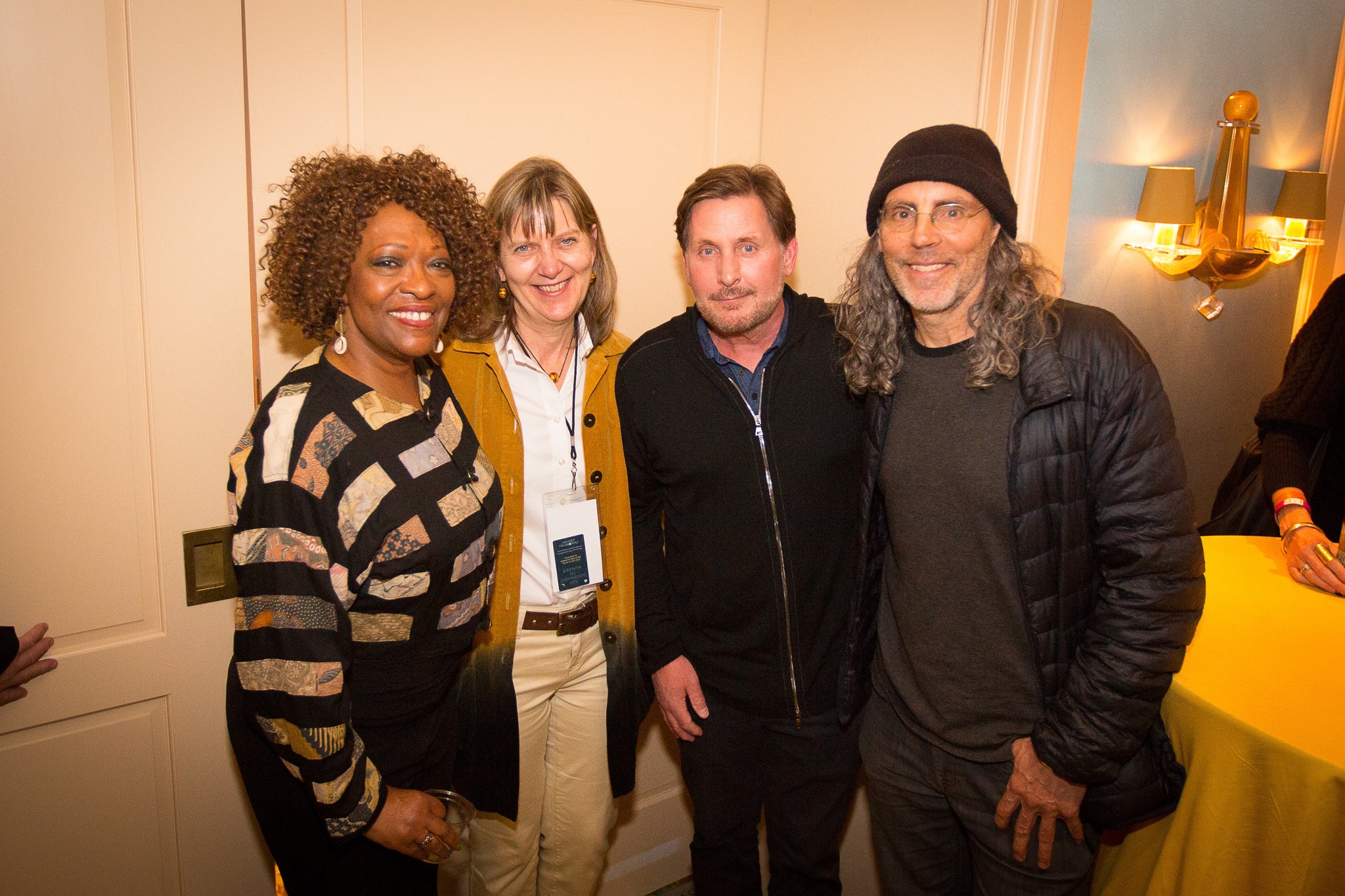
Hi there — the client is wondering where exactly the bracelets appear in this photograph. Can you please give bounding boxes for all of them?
[1280,523,1325,556]
[1274,497,1311,524]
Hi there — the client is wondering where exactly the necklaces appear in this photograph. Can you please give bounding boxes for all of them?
[518,331,576,382]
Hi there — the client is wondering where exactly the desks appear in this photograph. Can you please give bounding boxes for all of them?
[1093,534,1345,896]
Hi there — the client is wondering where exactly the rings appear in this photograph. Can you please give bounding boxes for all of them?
[1315,544,1334,565]
[1300,564,1312,577]
[421,834,433,848]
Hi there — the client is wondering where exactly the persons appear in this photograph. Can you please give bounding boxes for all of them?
[1193,272,1345,596]
[446,154,654,896]
[0,623,58,706]
[225,149,506,896]
[830,124,1205,896]
[613,162,880,896]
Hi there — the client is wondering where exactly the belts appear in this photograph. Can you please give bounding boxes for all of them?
[522,597,598,638]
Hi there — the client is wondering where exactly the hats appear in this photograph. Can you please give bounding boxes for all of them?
[866,125,1019,242]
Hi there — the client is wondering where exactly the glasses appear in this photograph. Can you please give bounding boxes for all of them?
[878,203,984,233]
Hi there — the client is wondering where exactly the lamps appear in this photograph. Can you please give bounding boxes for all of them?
[1124,89,1327,320]
